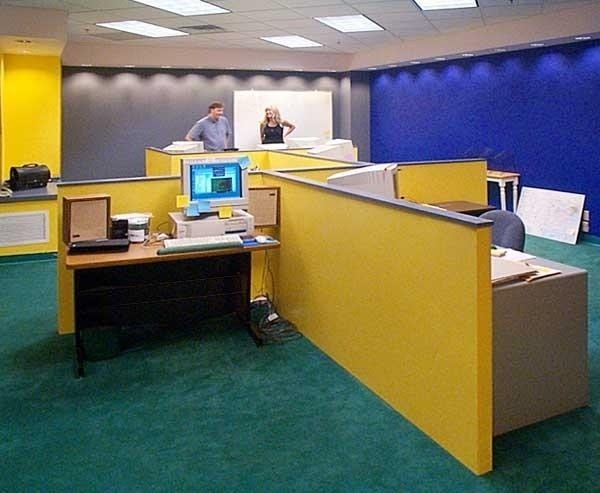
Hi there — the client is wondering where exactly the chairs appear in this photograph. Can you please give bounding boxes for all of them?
[478,210,525,253]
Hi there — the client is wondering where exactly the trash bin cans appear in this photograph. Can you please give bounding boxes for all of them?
[77,294,126,361]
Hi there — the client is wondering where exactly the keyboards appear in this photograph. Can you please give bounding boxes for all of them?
[164,234,243,248]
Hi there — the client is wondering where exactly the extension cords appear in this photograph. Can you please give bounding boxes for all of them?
[265,313,279,323]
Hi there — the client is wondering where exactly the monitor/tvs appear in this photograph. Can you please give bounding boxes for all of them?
[307,138,355,162]
[181,155,249,214]
[163,141,204,154]
[328,163,400,199]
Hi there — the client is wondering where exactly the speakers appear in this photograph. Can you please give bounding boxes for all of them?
[242,187,281,228]
[62,193,111,246]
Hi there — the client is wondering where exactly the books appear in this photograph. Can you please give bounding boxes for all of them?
[489,243,562,285]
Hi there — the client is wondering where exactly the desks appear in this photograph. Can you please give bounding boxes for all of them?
[64,228,280,378]
[486,169,521,213]
[0,181,58,256]
[490,243,589,437]
[430,200,497,216]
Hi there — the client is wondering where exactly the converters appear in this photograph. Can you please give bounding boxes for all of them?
[250,329,263,346]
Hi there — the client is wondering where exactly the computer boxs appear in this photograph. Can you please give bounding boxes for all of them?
[167,209,254,238]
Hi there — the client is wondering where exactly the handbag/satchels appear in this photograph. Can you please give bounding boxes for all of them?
[11,164,50,190]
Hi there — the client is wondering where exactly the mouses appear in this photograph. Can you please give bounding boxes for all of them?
[256,235,266,244]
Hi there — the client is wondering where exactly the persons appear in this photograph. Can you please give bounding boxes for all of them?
[258,104,296,143]
[184,100,232,153]
